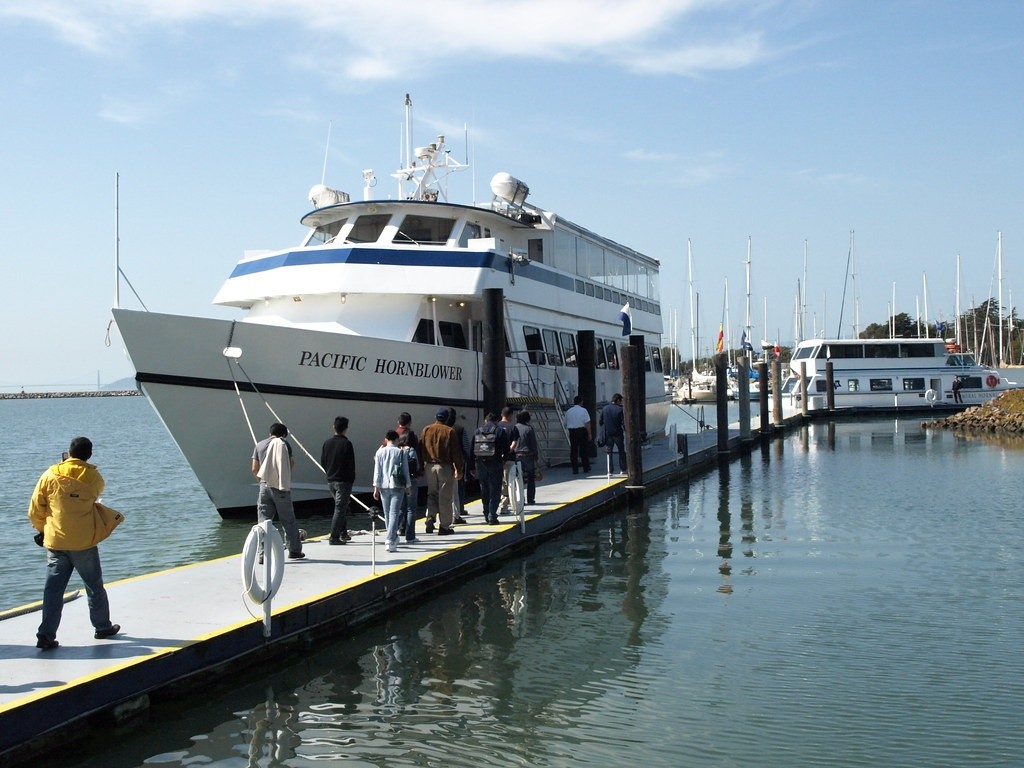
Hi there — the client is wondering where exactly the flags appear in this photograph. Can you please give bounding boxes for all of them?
[740,331,753,351]
[616,303,632,337]
[761,340,774,350]
[773,343,781,359]
[715,325,724,355]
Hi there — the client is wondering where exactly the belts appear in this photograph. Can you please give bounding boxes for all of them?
[427,461,444,464]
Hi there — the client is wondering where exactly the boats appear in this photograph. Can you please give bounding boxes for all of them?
[105,91,664,523]
[780,330,1024,419]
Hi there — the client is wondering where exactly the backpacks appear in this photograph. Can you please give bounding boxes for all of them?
[474,426,498,460]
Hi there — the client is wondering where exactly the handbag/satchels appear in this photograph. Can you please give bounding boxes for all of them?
[594,424,607,448]
[408,459,418,480]
[524,458,543,482]
[391,462,407,487]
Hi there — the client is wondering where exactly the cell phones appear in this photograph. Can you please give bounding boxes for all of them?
[62,452,69,462]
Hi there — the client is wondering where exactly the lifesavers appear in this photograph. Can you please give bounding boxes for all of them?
[986,375,997,387]
[241,522,286,606]
[925,389,937,402]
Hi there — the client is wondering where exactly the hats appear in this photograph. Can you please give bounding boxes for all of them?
[435,407,448,420]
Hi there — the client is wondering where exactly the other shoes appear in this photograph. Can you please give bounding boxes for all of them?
[342,533,351,539]
[288,552,306,559]
[425,522,434,533]
[258,555,264,564]
[407,537,421,544]
[460,509,467,515]
[384,539,397,552]
[485,517,499,525]
[572,471,578,474]
[585,469,591,472]
[398,528,406,536]
[497,508,513,515]
[329,537,346,544]
[526,500,535,504]
[453,516,466,524]
[437,527,455,535]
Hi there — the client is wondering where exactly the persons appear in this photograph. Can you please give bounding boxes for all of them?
[494,406,520,515]
[27,437,124,650]
[252,423,305,564]
[512,409,539,506]
[952,375,963,404]
[598,393,627,477]
[469,412,510,525]
[321,417,356,545]
[564,395,592,474]
[372,406,469,552]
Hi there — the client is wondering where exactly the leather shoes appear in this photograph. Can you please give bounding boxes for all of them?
[35,638,59,649]
[94,624,120,639]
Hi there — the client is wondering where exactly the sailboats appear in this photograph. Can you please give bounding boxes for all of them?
[663,235,1024,405]
